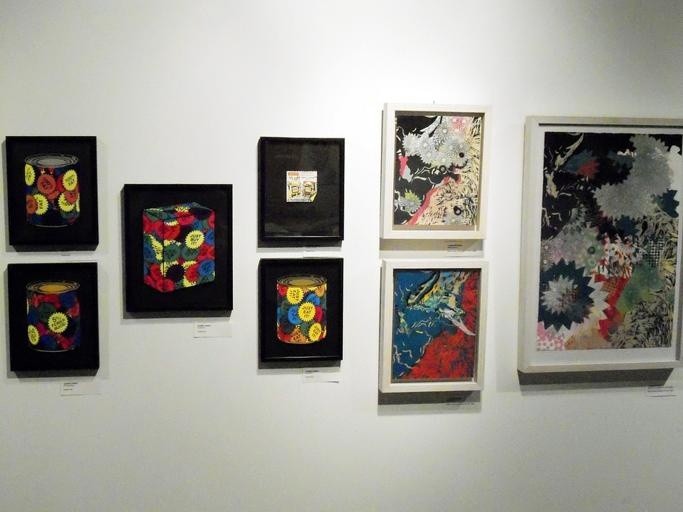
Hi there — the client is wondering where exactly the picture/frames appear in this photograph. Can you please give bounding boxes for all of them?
[253,136,344,368]
[3,134,99,378]
[118,181,232,314]
[374,102,492,398]
[515,114,681,376]
